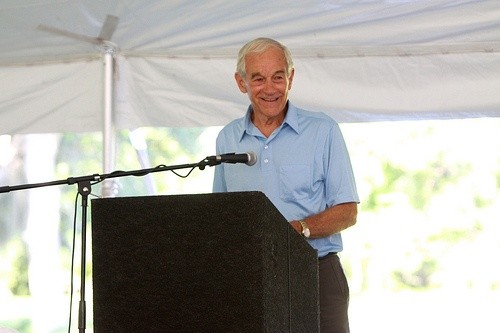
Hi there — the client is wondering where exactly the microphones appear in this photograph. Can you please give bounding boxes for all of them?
[207,152,257,166]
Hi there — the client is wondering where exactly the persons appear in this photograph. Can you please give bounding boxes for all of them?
[212,37,361,333]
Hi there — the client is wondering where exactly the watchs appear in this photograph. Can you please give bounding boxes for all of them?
[298,219,311,239]
